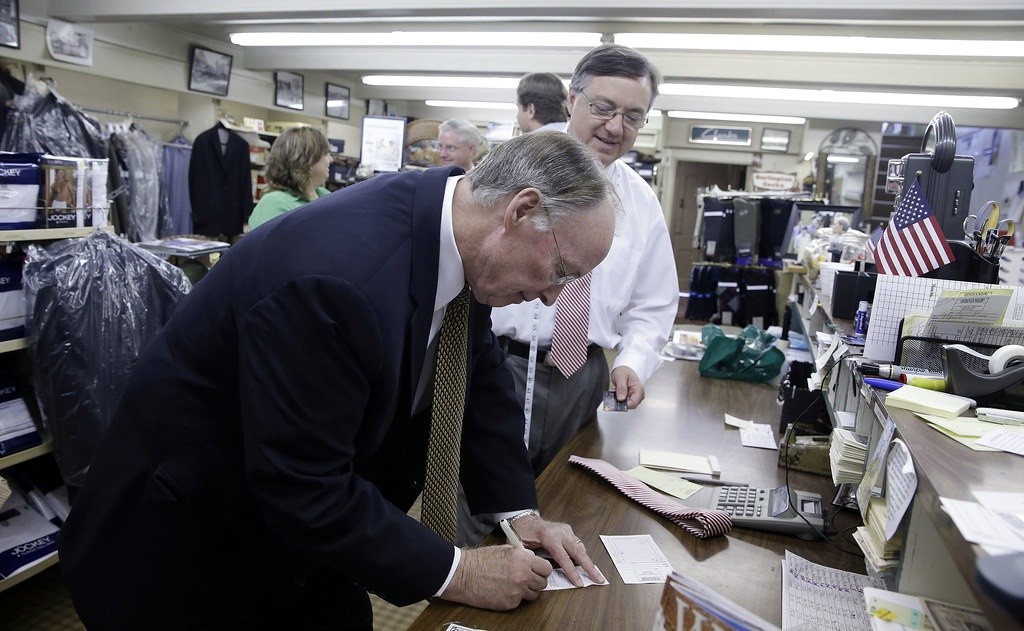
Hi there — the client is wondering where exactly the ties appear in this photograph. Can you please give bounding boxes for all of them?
[420,279,471,546]
[568,453,732,537]
[550,269,592,379]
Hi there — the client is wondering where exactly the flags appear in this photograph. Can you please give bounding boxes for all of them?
[874,178,954,279]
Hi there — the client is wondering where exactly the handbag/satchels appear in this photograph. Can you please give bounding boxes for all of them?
[698,335,785,382]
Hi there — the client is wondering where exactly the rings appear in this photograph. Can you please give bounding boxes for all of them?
[576,539,581,544]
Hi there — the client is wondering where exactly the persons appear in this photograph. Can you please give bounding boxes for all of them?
[455,46,680,549]
[436,118,490,174]
[54,133,618,631]
[246,127,334,233]
[517,72,571,138]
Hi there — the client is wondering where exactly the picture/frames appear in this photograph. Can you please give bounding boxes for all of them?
[273,72,304,111]
[324,83,351,120]
[0,0,21,48]
[189,47,233,96]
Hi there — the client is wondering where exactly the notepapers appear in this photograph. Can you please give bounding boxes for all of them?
[885,385,970,419]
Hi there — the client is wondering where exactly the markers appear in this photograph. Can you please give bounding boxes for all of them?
[861,363,946,391]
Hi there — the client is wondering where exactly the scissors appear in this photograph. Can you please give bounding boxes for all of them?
[974,201,1015,249]
[962,215,989,247]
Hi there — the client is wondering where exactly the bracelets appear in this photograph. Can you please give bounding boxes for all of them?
[510,511,537,524]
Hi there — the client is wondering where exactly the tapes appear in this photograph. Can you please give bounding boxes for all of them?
[948,344,1024,375]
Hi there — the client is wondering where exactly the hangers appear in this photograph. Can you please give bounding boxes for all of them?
[41,76,142,131]
[215,109,232,132]
[175,121,189,142]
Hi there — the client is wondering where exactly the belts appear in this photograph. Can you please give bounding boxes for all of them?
[496,334,602,368]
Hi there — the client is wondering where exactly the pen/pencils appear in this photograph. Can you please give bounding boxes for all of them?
[499,518,526,549]
[682,476,749,487]
[976,407,1024,426]
[976,229,1011,264]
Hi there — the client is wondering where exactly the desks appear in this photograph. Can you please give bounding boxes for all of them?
[408,262,1022,631]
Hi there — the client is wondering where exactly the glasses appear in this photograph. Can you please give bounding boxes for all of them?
[579,89,649,130]
[541,200,576,285]
